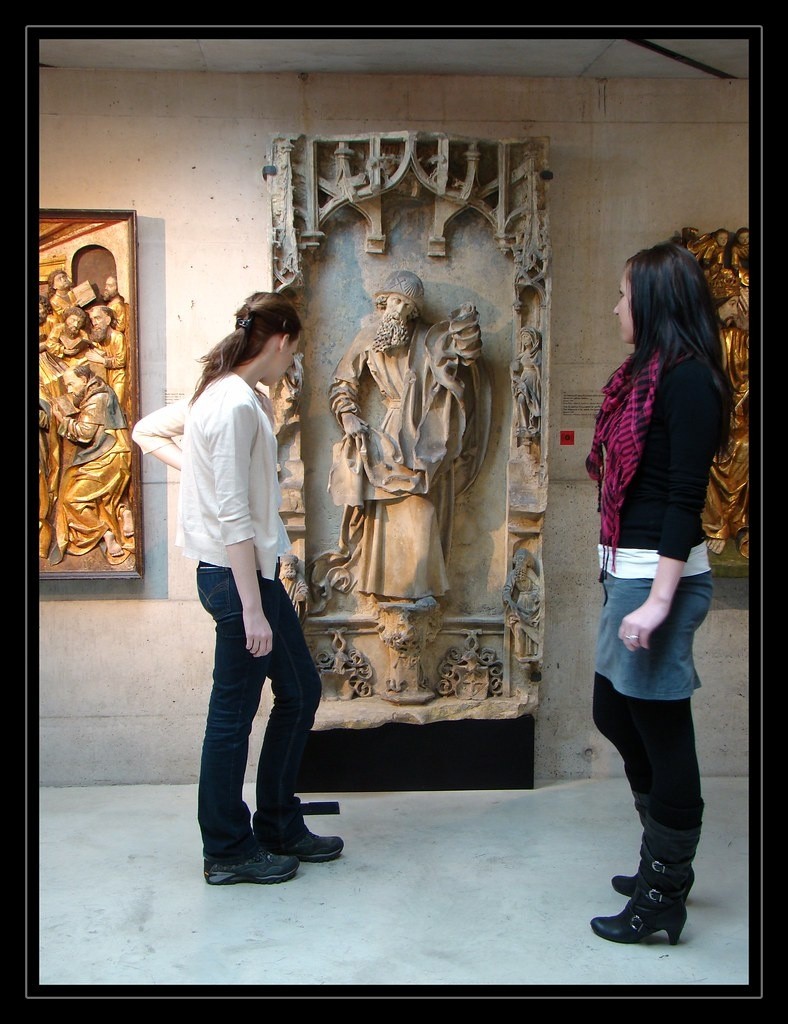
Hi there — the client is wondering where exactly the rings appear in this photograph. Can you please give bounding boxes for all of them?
[625,636,630,639]
[630,635,639,639]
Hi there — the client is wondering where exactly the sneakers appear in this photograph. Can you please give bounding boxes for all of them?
[281,831,344,863]
[204,847,300,886]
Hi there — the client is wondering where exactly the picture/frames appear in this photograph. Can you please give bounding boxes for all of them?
[40,207,145,582]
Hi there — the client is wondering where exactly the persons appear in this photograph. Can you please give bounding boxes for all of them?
[278,555,310,628]
[706,265,748,555]
[38,270,136,566]
[585,241,736,945]
[510,325,542,444]
[133,291,344,885]
[330,270,481,604]
[502,547,541,657]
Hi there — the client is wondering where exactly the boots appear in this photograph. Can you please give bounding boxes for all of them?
[590,813,701,945]
[611,790,695,904]
